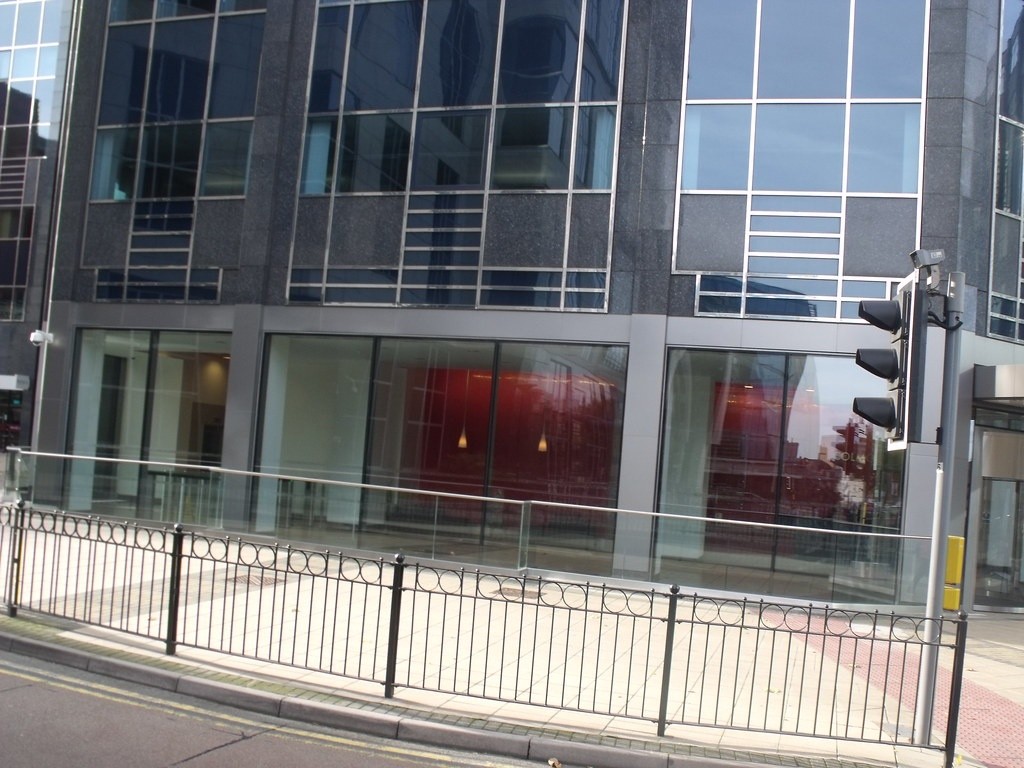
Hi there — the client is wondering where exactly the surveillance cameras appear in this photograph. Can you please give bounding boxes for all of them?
[29,332,43,346]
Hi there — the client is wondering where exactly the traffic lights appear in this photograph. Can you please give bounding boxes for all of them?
[852,270,917,450]
